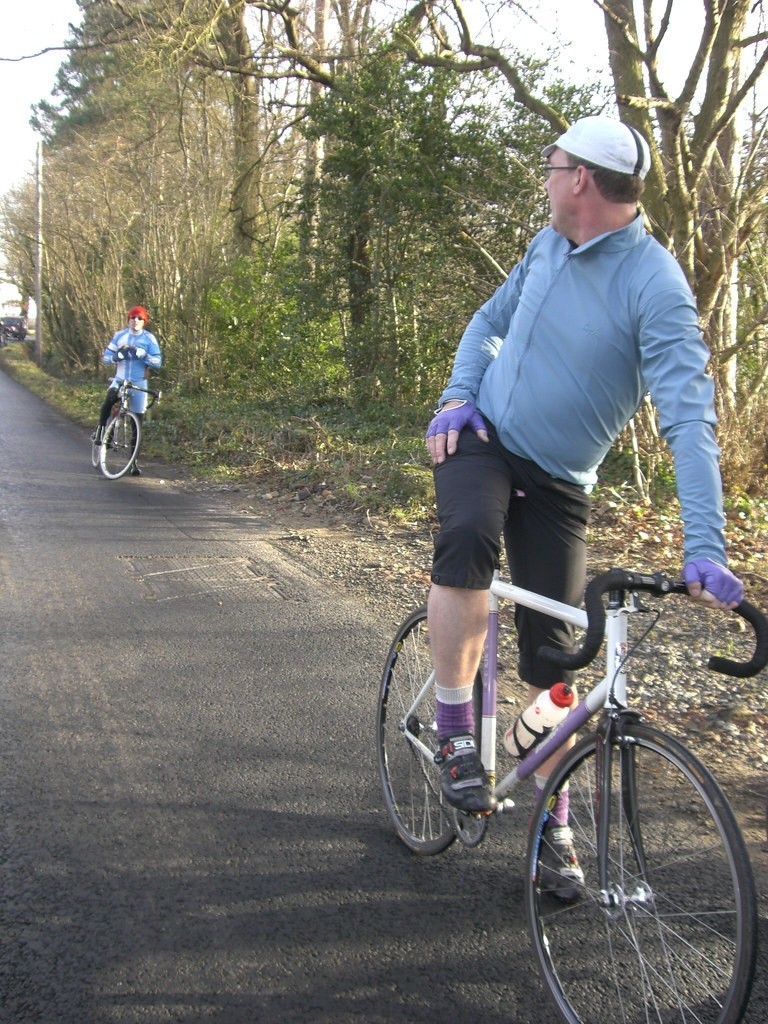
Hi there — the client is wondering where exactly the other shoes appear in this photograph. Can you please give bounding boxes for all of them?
[92,425,105,445]
[130,458,142,476]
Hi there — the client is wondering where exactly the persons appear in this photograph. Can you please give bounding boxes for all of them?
[94,305,163,474]
[427,118,748,902]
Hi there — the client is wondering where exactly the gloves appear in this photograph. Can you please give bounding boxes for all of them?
[112,345,129,362]
[127,346,148,360]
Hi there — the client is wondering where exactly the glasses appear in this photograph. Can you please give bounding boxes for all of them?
[542,163,599,177]
[129,316,145,320]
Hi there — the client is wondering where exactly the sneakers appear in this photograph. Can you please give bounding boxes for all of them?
[435,730,497,812]
[539,825,585,903]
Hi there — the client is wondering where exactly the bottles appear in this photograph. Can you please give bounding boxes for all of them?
[503,682,574,756]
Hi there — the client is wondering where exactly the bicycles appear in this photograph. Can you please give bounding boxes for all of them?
[90,379,163,479]
[376,484,768,1024]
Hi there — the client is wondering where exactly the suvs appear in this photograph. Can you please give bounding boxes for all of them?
[1,316,28,342]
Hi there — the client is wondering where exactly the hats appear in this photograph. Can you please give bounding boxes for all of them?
[540,116,651,180]
[128,306,148,324]
